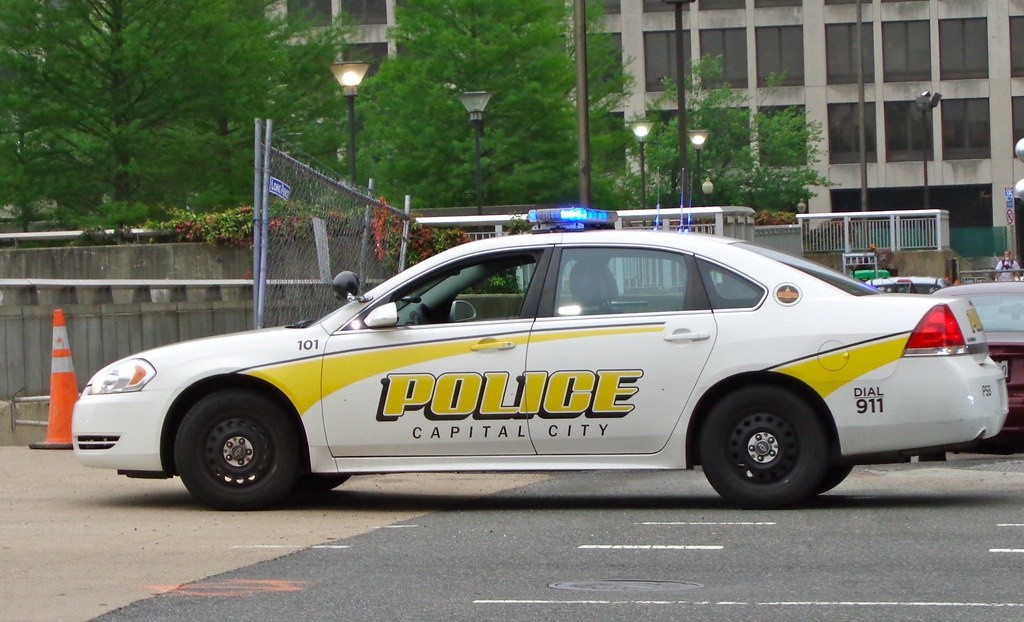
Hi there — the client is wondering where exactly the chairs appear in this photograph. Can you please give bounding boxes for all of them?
[568,261,619,314]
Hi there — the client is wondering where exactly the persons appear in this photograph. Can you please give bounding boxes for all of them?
[995,250,1020,282]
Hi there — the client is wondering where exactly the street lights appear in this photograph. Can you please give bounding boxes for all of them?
[626,119,656,227]
[685,129,708,205]
[916,90,944,209]
[328,60,372,184]
[454,89,496,213]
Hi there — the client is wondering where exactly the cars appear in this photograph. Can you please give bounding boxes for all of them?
[71,208,1008,511]
[863,277,956,298]
[929,278,1024,446]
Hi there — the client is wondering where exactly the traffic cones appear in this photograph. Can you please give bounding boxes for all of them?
[28,309,84,451]
